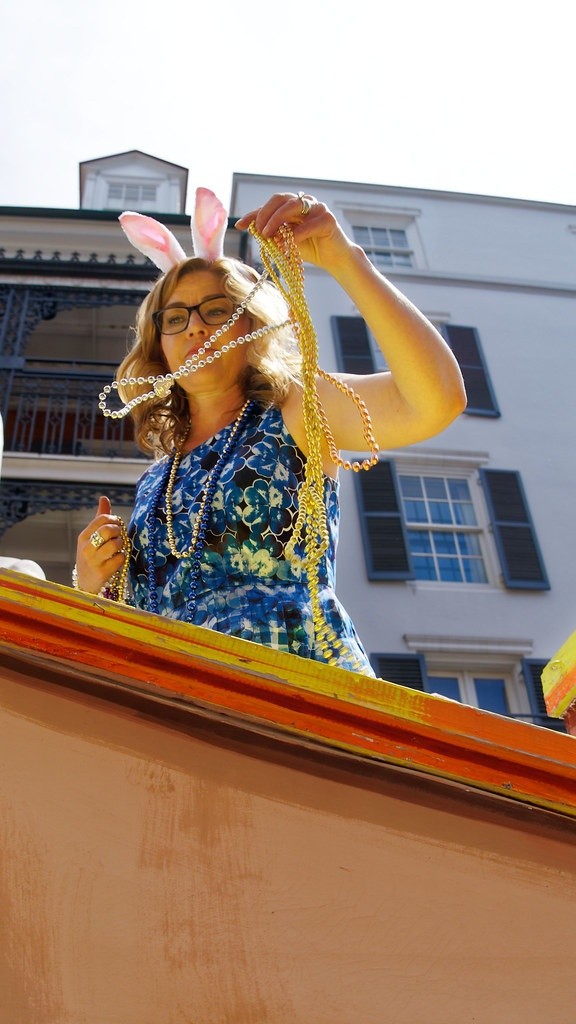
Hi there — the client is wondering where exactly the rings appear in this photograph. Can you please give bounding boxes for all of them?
[89,531,105,548]
[295,190,306,199]
[300,198,311,215]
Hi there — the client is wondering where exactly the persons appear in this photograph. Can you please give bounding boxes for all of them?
[64,190,470,688]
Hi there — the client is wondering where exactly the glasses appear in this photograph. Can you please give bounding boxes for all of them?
[151,294,245,334]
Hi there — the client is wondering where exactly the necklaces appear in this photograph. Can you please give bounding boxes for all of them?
[143,400,256,624]
[159,395,250,558]
